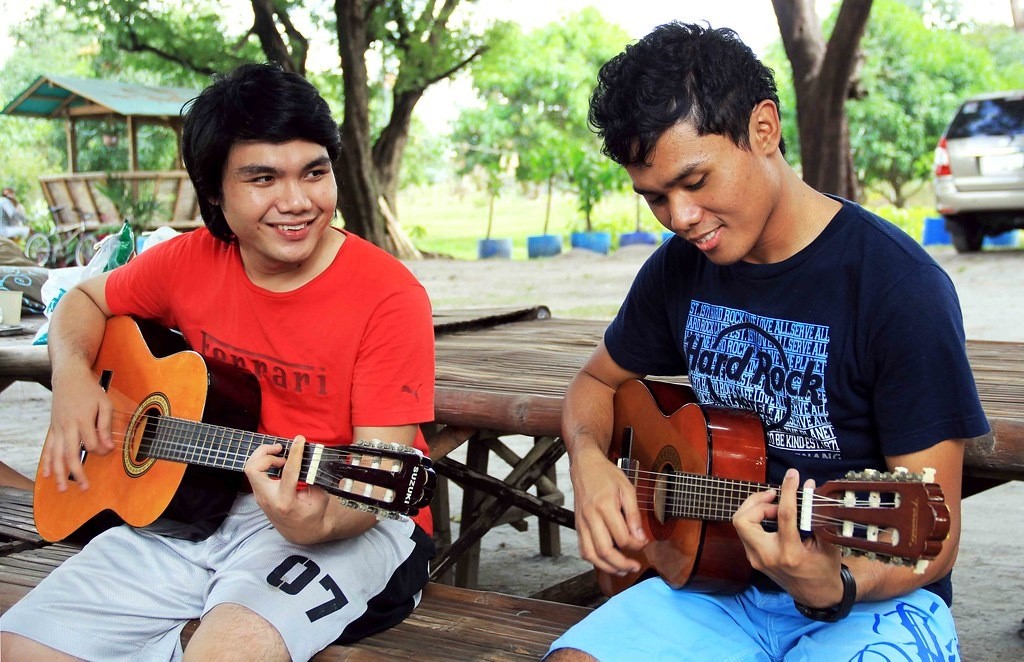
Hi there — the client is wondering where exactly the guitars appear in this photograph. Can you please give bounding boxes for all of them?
[590,376,951,597]
[30,311,439,551]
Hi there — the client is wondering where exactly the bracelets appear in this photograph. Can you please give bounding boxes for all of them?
[793,563,856,622]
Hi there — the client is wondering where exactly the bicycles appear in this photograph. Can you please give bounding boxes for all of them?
[24,206,100,267]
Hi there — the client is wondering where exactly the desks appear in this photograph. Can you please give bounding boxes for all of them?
[3,293,1023,498]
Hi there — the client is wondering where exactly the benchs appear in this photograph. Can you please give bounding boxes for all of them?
[0,465,609,662]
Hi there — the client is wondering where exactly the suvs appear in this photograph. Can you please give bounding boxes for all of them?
[932,92,1024,253]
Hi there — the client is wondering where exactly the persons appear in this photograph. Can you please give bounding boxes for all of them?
[0,188,34,240]
[541,21,991,662]
[0,63,437,662]
